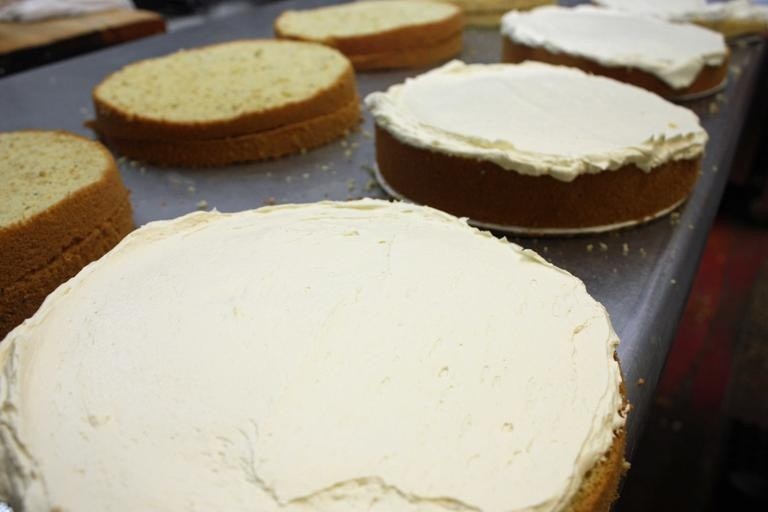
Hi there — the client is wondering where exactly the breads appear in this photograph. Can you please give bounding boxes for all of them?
[456,0,555,30]
[93,39,358,166]
[0,129,135,341]
[274,0,463,70]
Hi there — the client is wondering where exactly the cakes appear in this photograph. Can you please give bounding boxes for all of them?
[501,4,731,96]
[364,60,709,228]
[590,0,768,40]
[0,199,631,512]
